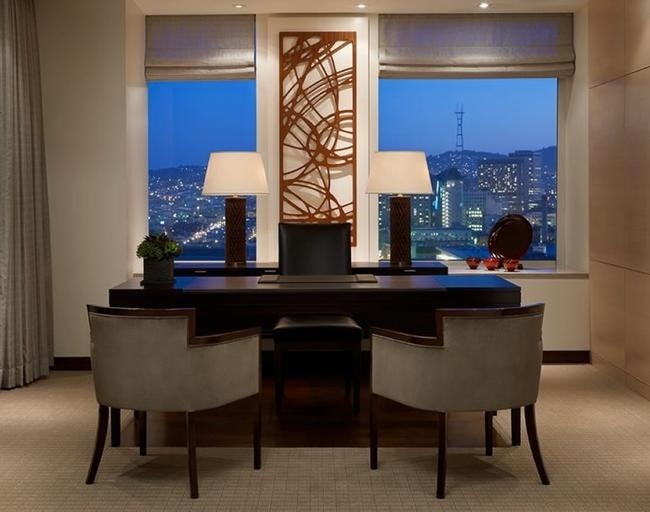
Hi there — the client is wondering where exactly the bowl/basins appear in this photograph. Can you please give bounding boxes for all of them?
[484,258,500,270]
[466,256,482,268]
[502,259,519,271]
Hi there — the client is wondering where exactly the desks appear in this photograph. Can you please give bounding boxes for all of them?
[108,260,523,447]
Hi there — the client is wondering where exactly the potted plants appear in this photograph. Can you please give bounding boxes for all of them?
[137,233,184,283]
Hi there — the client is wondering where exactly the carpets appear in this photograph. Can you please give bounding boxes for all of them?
[120,349,510,447]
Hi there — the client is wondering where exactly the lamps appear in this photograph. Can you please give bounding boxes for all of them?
[365,151,434,265]
[201,152,270,265]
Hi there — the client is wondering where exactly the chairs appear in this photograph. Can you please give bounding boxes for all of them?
[365,302,550,498]
[85,303,263,499]
[273,222,363,417]
